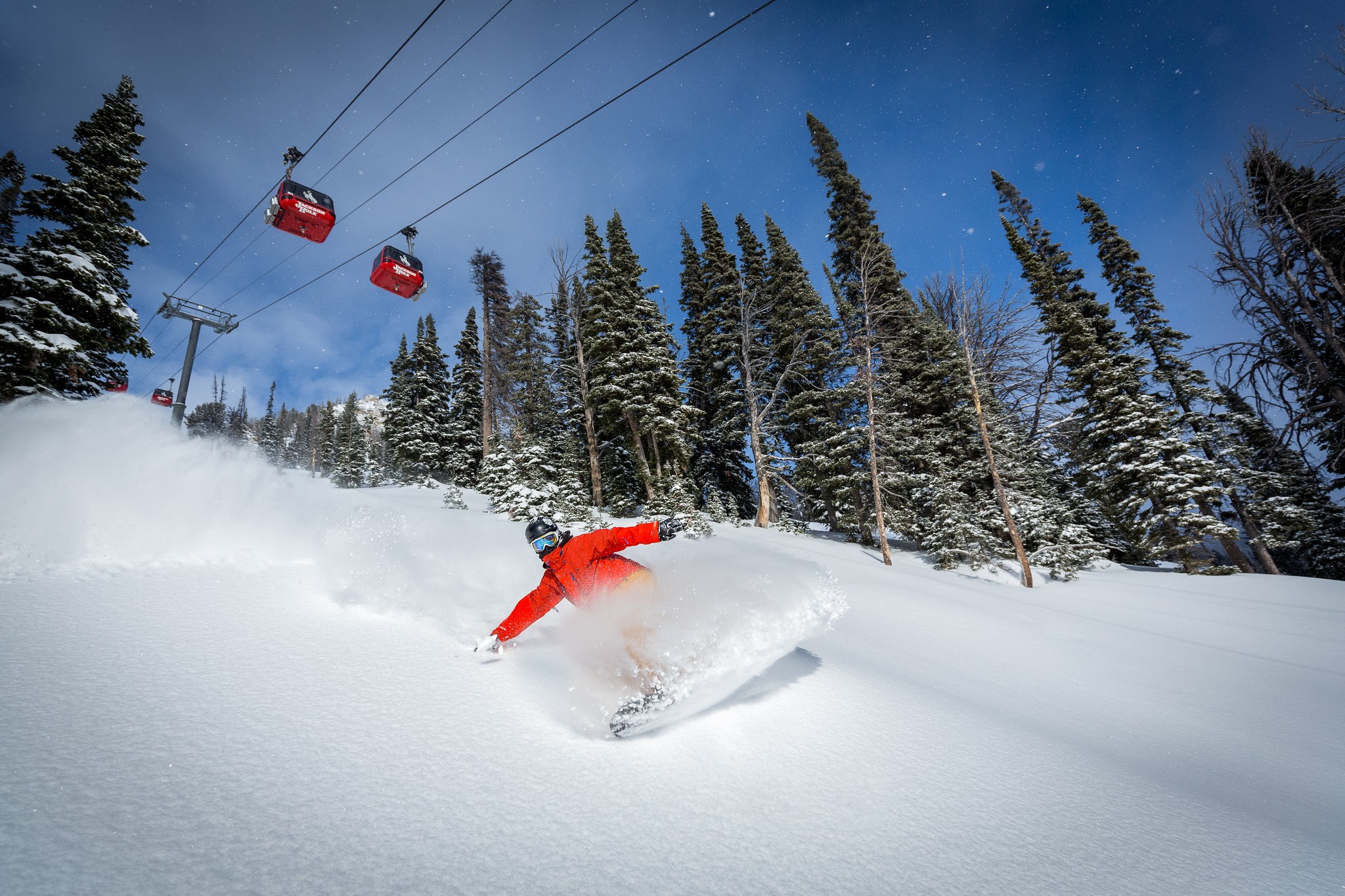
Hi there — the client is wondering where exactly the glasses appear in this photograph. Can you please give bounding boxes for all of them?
[530,532,559,552]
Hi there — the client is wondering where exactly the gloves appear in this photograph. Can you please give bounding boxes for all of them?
[657,517,683,541]
[474,634,499,652]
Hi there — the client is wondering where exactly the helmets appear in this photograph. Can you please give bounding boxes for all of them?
[524,517,560,554]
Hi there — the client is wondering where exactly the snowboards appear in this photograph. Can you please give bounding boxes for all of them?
[603,648,822,738]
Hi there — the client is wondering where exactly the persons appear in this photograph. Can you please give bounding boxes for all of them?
[474,513,692,731]
